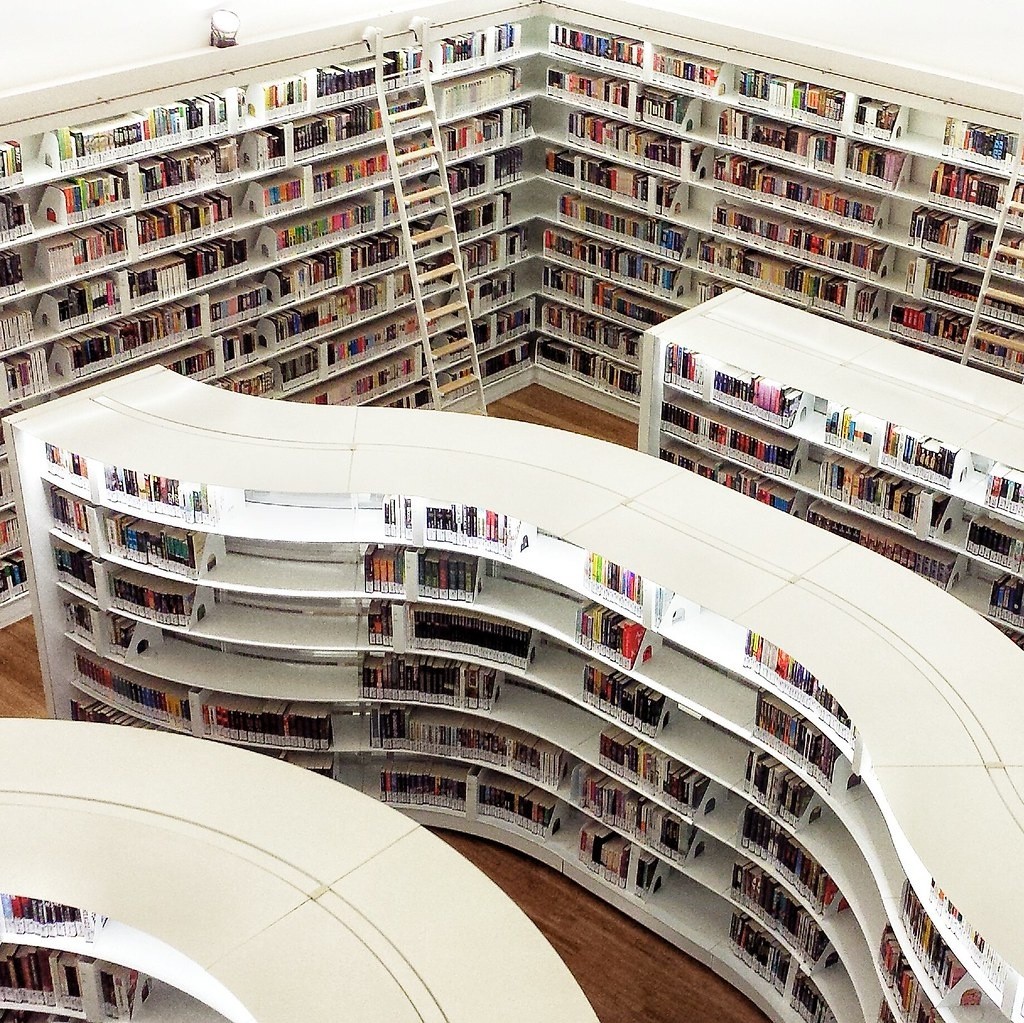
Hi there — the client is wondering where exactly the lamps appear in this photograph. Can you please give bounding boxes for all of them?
[209,9,241,49]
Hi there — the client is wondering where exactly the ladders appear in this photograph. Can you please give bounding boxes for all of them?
[363,15,491,417]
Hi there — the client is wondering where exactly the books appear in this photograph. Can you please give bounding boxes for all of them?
[0,22,1024,1021]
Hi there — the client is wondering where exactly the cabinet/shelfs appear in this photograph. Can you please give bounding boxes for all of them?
[0,718,608,1023]
[535,0,1024,427]
[638,289,1024,652]
[1,365,1024,1023]
[0,0,542,631]
